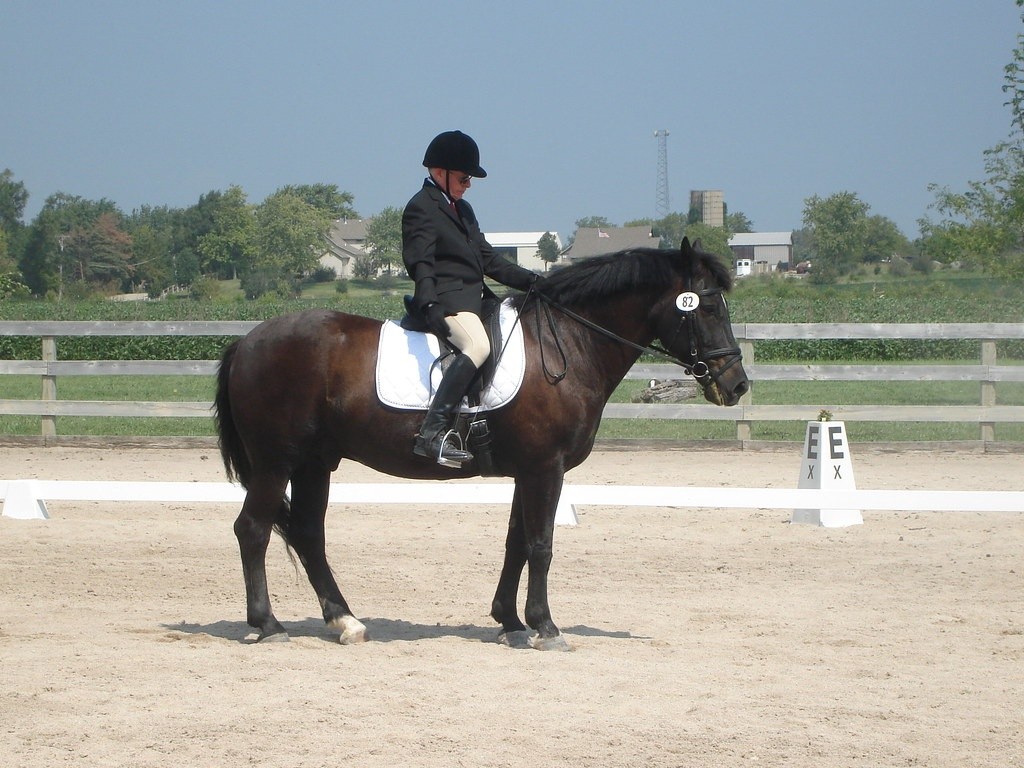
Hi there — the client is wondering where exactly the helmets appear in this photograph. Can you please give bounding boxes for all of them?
[422,130,487,178]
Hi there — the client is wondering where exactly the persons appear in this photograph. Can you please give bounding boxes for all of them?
[402,130,547,461]
[777,261,783,271]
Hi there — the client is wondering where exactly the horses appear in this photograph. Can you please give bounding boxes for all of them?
[208,237,751,651]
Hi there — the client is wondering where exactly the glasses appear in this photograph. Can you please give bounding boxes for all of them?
[449,171,473,183]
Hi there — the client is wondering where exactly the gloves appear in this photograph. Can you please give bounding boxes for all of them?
[422,301,457,339]
[527,274,545,291]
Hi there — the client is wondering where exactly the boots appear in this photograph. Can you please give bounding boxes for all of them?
[413,354,478,461]
